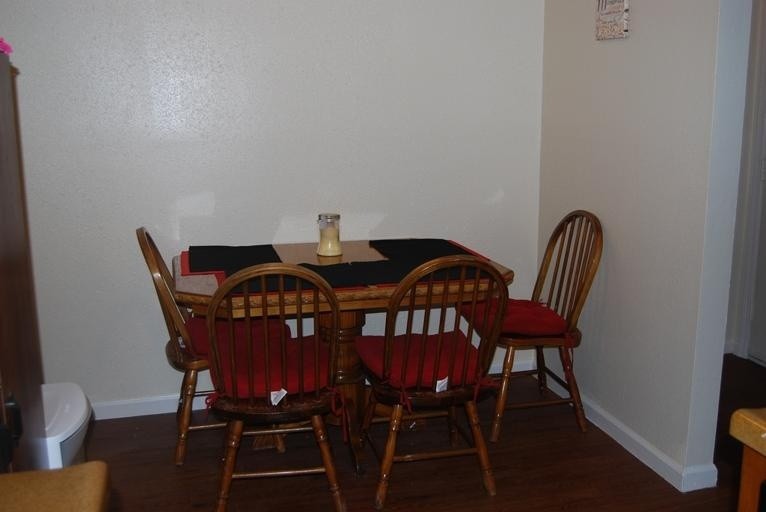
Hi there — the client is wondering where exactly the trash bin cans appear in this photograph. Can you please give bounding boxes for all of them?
[42,382,92,469]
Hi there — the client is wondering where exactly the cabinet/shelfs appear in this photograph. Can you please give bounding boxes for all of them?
[0,52,49,474]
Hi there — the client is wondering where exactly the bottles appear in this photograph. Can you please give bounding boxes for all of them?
[317,212,343,257]
